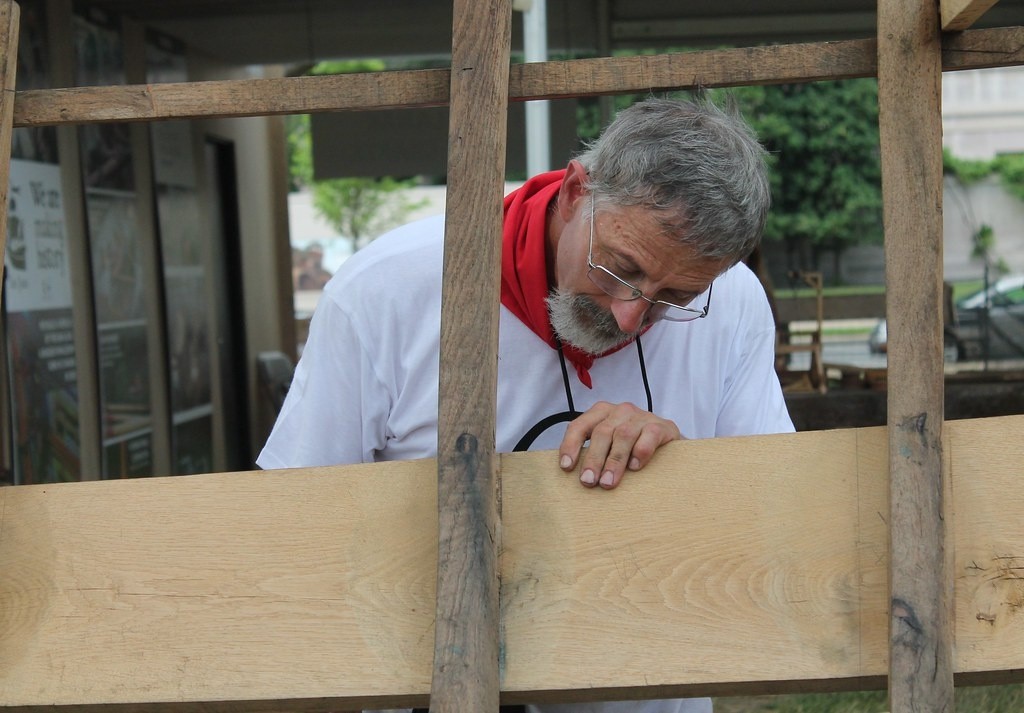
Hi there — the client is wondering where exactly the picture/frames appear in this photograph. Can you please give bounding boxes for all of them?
[73,15,138,201]
[144,65,208,194]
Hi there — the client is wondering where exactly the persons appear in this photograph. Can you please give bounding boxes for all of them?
[255,90,796,713]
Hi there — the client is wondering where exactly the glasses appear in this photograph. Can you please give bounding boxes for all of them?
[586,170,713,323]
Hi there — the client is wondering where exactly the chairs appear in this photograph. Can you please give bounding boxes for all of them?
[257,351,295,416]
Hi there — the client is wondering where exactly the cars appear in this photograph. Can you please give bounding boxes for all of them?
[867,274,1024,368]
